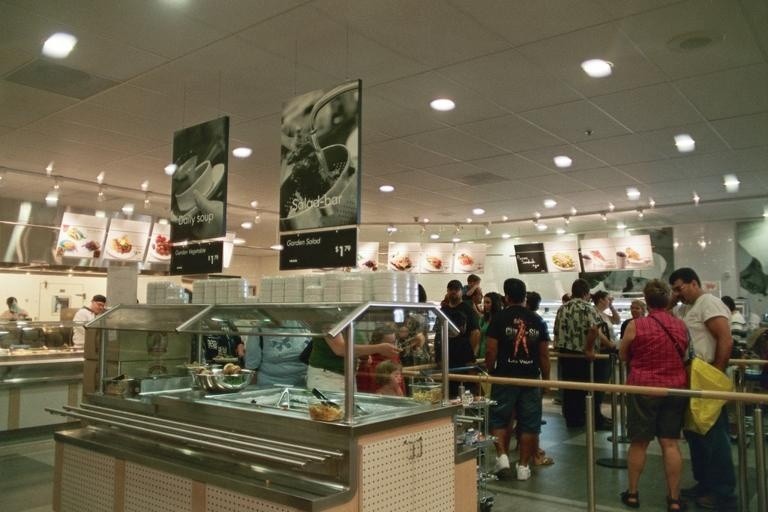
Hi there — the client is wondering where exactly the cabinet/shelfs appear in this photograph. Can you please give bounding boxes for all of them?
[42,301,498,512]
[0,321,84,431]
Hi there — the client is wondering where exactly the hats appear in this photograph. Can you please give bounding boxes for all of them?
[468,275,481,282]
[93,295,106,302]
[447,280,463,289]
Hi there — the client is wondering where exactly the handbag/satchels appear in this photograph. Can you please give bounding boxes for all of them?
[600,322,610,346]
[299,340,313,365]
[682,328,735,436]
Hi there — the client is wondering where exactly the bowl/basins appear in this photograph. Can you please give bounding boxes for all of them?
[307,396,347,422]
[196,369,256,392]
[102,377,141,397]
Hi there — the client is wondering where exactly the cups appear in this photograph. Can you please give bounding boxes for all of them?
[174,160,213,212]
[582,255,593,271]
[616,251,627,269]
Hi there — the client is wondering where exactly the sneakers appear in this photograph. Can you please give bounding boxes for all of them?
[596,421,613,430]
[696,491,733,509]
[602,417,612,423]
[515,463,531,480]
[551,397,562,405]
[492,454,510,476]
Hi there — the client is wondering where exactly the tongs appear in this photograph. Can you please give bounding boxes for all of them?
[311,387,339,410]
[277,387,290,408]
[396,336,422,355]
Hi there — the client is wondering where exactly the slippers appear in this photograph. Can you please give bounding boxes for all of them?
[532,458,554,466]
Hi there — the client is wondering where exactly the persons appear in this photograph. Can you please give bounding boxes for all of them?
[399,274,553,482]
[0,296,29,319]
[199,320,407,398]
[554,267,767,511]
[71,294,106,348]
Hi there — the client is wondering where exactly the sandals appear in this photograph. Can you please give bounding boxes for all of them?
[620,490,639,507]
[666,496,687,512]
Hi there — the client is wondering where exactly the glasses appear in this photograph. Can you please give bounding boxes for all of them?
[672,283,687,292]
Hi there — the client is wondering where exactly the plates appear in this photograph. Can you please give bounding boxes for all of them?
[456,253,475,272]
[151,249,171,260]
[629,257,648,264]
[212,357,238,362]
[423,256,442,272]
[389,262,415,271]
[106,244,136,259]
[551,256,576,270]
[171,163,225,223]
